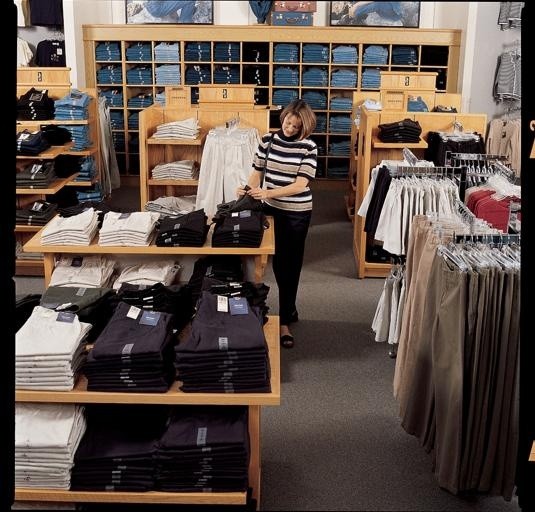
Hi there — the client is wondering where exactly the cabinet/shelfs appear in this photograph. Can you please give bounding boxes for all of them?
[280,27,486,279]
[344,117,361,218]
[16,27,280,512]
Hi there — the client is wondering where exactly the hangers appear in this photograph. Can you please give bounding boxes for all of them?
[487,153,520,276]
[491,38,523,131]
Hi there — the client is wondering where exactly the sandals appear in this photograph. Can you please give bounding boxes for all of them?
[281,310,298,348]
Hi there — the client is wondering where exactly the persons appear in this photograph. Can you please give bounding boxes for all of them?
[234,98,319,350]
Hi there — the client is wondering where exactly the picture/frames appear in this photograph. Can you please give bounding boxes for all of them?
[123,0,215,27]
[329,1,421,29]
